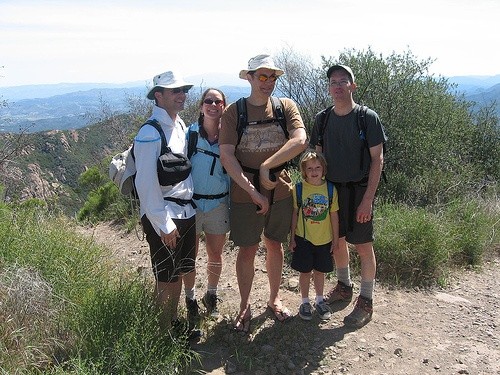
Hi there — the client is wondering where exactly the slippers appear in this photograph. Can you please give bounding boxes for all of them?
[267,300,291,323]
[234,304,251,332]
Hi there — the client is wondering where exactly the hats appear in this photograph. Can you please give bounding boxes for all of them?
[109,146,136,196]
[327,64,354,83]
[239,55,285,80]
[146,71,194,100]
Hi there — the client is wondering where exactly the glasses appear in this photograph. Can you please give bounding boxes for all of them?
[328,80,350,85]
[203,99,224,105]
[171,88,188,93]
[253,74,277,82]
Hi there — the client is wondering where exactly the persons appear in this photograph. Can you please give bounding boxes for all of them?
[132,71,197,338]
[307,65,387,327]
[218,55,307,333]
[288,152,340,320]
[182,88,231,320]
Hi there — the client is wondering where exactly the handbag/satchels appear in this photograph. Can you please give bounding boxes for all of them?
[156,149,192,186]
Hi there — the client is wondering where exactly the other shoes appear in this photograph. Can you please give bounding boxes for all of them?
[172,318,201,341]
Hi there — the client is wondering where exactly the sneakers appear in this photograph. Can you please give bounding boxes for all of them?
[299,302,313,320]
[202,293,223,319]
[185,299,200,326]
[323,279,353,304]
[314,301,331,319]
[343,295,373,328]
